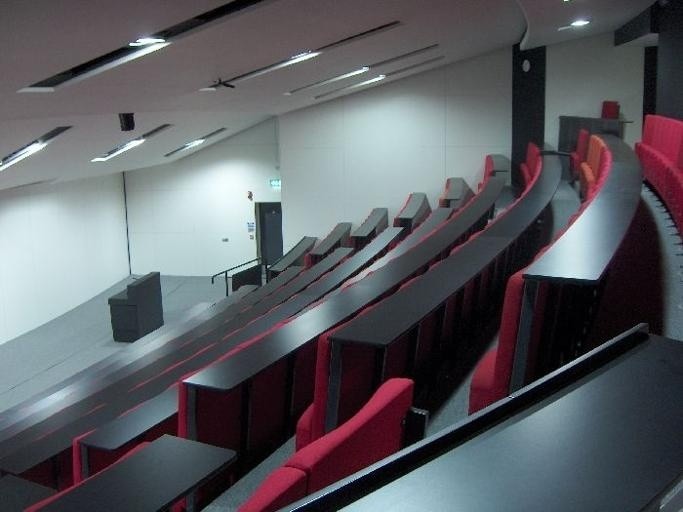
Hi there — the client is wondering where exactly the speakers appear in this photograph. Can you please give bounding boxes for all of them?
[118,113,136,131]
